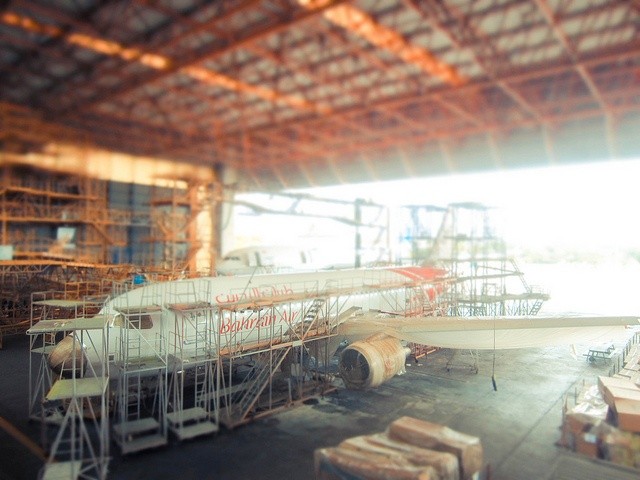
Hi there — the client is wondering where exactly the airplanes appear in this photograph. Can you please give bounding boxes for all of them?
[50,197,640,391]
